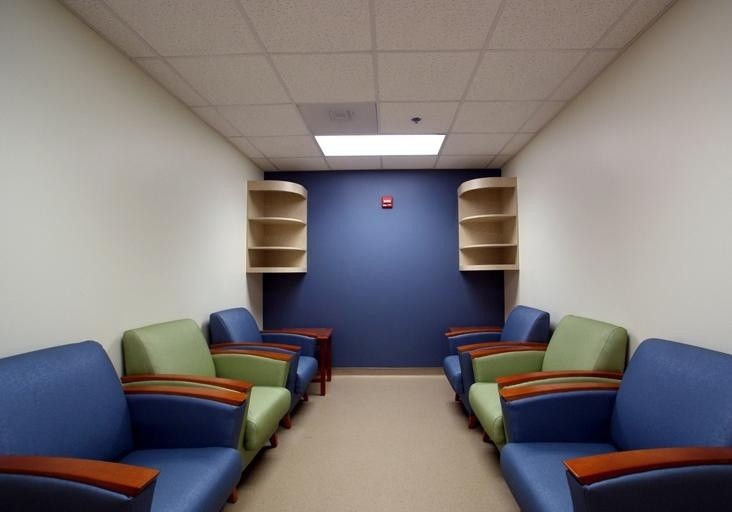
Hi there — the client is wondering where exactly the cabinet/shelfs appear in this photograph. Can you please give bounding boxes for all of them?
[458,176,518,272]
[246,180,309,274]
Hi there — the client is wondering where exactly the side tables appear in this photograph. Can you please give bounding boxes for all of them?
[270,328,334,396]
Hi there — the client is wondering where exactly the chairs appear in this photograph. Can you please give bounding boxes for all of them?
[496,335,732,511]
[0,340,251,512]
[119,317,294,468]
[204,307,322,403]
[444,305,552,407]
[466,312,630,457]
